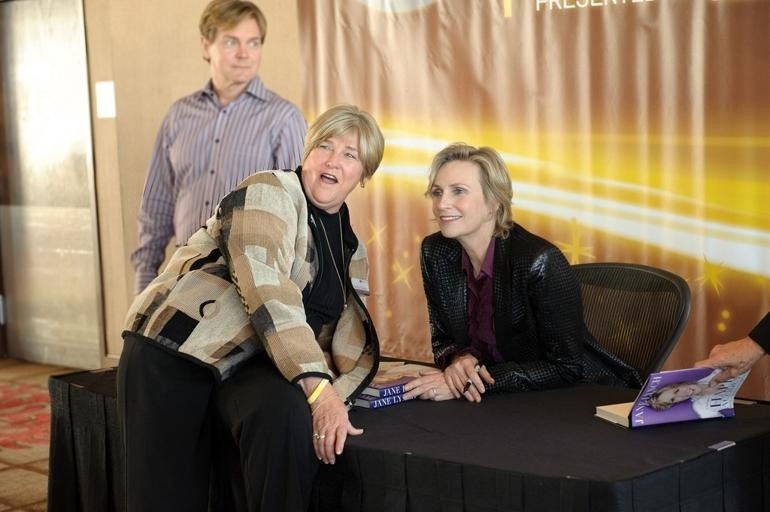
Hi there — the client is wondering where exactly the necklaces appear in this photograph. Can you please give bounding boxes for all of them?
[319,211,347,312]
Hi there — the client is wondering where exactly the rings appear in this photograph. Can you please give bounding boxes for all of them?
[313,432,326,440]
[434,388,436,395]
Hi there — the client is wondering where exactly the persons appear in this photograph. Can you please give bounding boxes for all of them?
[694,311,770,388]
[402,141,643,403]
[129,0,309,301]
[646,369,751,419]
[115,105,386,512]
[372,364,440,385]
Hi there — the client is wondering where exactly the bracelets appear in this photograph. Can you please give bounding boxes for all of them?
[307,378,329,404]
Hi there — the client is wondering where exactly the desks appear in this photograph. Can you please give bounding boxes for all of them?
[47,354,770,512]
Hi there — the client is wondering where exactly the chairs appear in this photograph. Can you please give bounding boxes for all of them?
[572,263,690,387]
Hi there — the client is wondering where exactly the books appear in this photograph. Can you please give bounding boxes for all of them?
[361,363,442,398]
[354,394,417,410]
[593,367,753,429]
